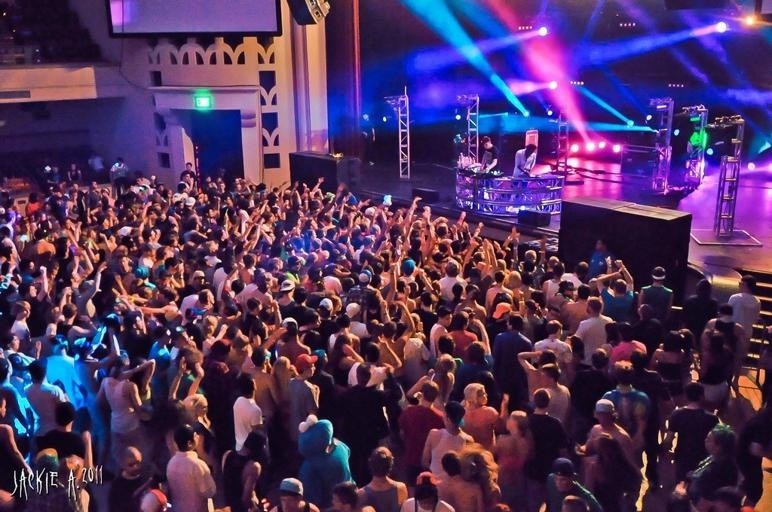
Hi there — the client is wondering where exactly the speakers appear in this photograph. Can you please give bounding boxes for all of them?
[288,152,350,192]
[519,209,551,227]
[288,0,331,26]
[413,188,439,203]
[557,198,693,301]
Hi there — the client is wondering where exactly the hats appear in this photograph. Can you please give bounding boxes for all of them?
[651,266,667,280]
[458,382,485,407]
[558,279,578,292]
[416,469,446,486]
[525,249,538,260]
[140,489,174,512]
[34,446,61,482]
[595,398,616,415]
[443,399,467,429]
[28,182,489,385]
[480,134,491,144]
[248,428,270,452]
[491,301,512,320]
[550,457,579,477]
[279,476,304,496]
[174,421,201,444]
[54,401,79,427]
[546,255,560,266]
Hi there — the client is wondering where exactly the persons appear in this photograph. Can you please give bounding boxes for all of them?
[2,136,772,512]
